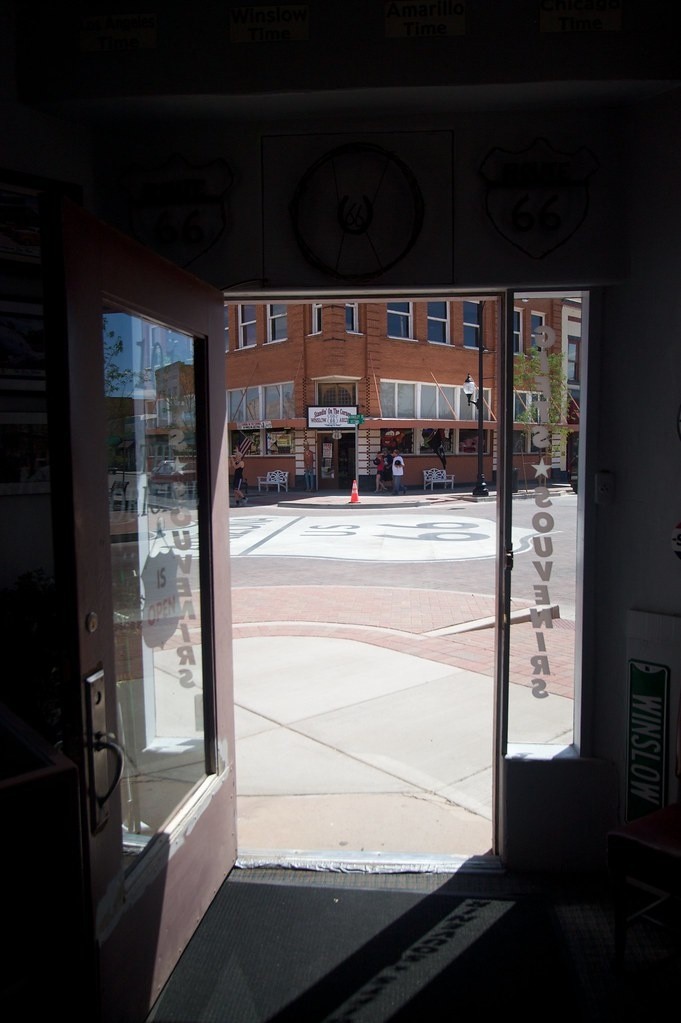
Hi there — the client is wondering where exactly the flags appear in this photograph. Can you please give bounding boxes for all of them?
[266,433,277,452]
[426,428,447,470]
[238,437,253,456]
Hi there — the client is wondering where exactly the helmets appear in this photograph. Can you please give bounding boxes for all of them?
[395,460,401,465]
[373,458,380,465]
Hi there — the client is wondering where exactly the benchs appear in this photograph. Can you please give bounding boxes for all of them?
[421,468,455,492]
[257,469,289,493]
[109,479,130,512]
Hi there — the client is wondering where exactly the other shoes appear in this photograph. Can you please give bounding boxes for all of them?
[403,487,407,495]
[373,491,379,494]
[383,488,389,494]
[391,491,399,495]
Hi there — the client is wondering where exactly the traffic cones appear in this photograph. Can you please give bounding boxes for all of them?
[348,480,361,503]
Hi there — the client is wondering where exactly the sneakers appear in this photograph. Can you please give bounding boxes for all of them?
[238,503,243,506]
[243,497,248,504]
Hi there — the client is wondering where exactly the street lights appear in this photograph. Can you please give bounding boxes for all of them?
[463,373,489,497]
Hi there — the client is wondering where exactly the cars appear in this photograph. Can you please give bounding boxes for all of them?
[150,461,197,497]
[569,453,578,494]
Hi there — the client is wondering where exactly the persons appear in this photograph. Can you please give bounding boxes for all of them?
[230,452,248,507]
[374,449,408,495]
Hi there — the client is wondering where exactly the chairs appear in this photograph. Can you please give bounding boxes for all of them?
[608,686,681,958]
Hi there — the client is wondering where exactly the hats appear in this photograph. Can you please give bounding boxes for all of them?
[394,450,399,455]
